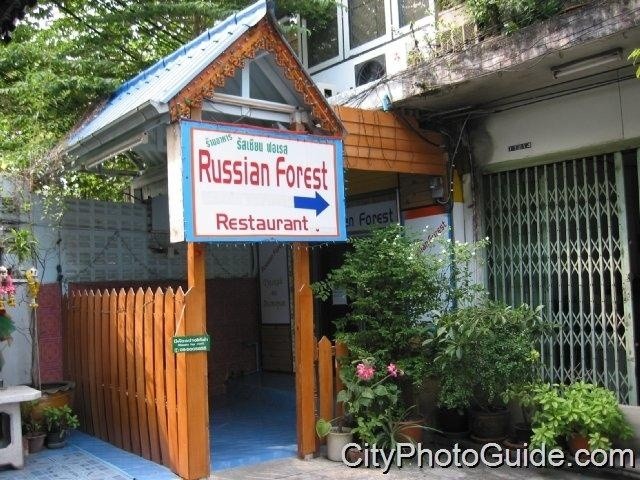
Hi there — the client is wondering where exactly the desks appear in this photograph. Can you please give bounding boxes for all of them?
[0,385,42,472]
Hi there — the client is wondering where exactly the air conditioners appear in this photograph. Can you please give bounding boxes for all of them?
[353,42,410,88]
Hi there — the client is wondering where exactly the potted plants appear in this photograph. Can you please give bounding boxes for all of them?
[1,184,81,459]
[306,219,635,463]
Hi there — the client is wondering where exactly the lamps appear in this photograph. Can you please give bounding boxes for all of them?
[84,130,149,171]
[551,47,625,81]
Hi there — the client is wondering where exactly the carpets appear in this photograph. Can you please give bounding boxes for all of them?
[0,443,137,480]
